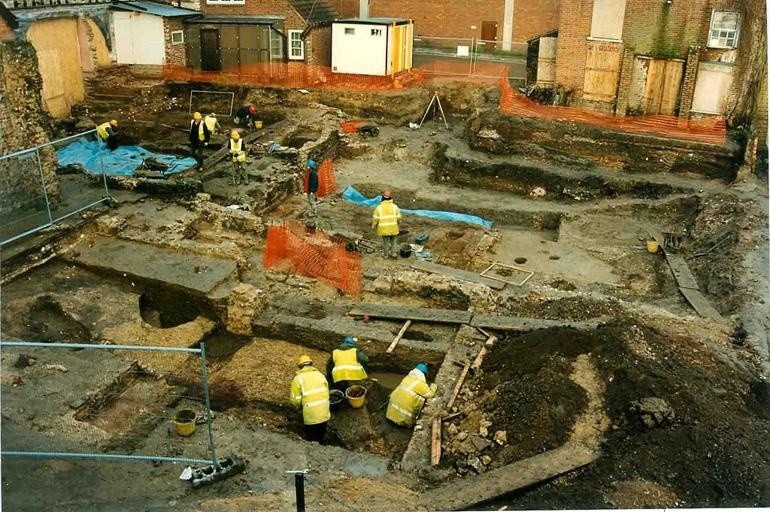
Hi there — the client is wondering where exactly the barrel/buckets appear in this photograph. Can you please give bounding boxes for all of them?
[647,242,658,252]
[345,385,367,407]
[329,389,344,410]
[173,408,196,436]
[254,121,263,128]
[400,243,412,258]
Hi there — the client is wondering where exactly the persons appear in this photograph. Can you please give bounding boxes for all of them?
[204,112,224,148]
[187,112,211,172]
[233,105,256,130]
[327,336,371,392]
[289,353,331,444]
[227,131,249,185]
[304,159,319,217]
[384,363,439,429]
[93,119,121,151]
[370,192,402,260]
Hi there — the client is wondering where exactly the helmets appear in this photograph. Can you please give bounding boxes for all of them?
[194,112,202,119]
[382,191,392,199]
[416,363,429,376]
[343,336,355,345]
[109,119,118,127]
[306,159,317,169]
[249,106,255,114]
[231,131,240,138]
[298,355,313,366]
